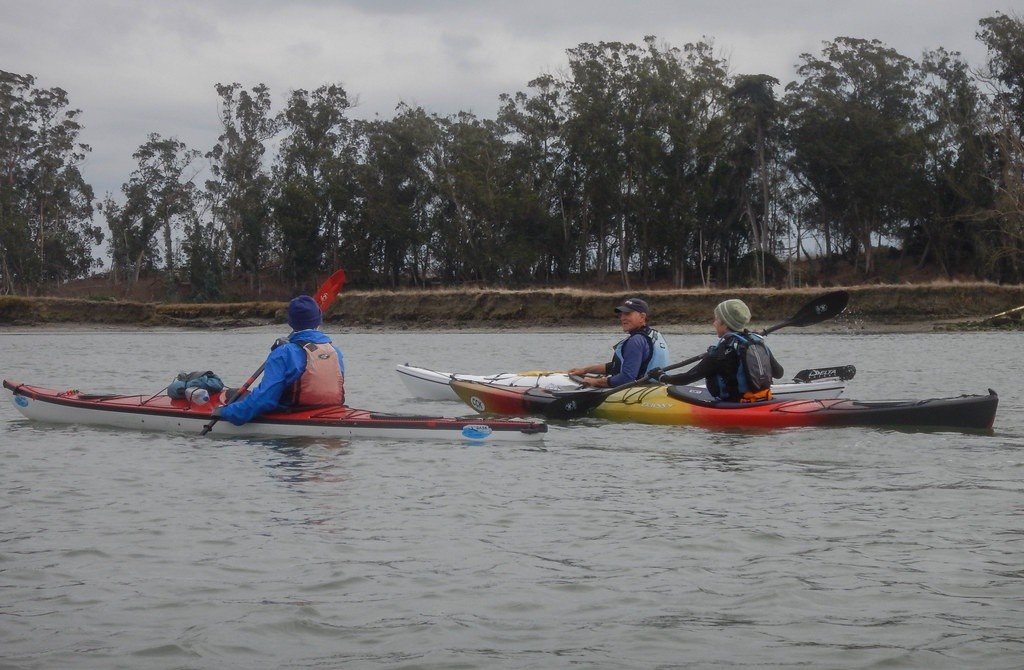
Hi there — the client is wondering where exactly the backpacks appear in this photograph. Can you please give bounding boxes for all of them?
[718,332,773,393]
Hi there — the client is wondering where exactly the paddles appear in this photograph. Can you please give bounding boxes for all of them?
[197,267,349,439]
[540,287,853,422]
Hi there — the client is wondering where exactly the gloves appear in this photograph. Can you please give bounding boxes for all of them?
[211,406,224,417]
[647,367,666,382]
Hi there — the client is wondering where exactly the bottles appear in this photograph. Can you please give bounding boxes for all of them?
[185,386,210,406]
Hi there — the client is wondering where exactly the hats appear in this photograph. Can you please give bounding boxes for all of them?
[287,296,323,329]
[614,298,649,315]
[715,299,751,332]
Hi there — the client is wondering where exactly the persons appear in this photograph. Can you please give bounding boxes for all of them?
[647,299,784,403]
[211,295,345,426]
[568,297,668,389]
